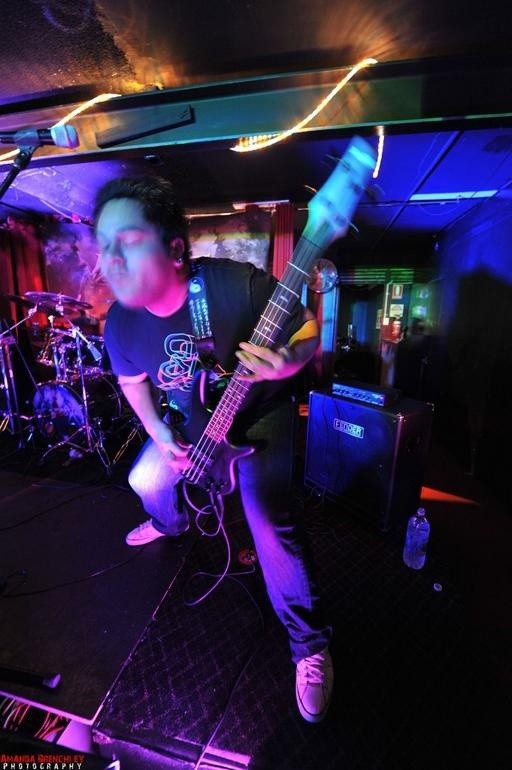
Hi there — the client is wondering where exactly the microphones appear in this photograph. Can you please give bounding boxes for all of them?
[0,123,81,150]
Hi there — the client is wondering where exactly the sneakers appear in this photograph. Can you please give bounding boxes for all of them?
[125,516,188,547]
[294,646,336,722]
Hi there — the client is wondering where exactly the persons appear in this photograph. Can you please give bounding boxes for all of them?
[92,174,335,724]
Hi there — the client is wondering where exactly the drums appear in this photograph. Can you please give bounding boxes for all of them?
[30,375,122,447]
[35,328,109,375]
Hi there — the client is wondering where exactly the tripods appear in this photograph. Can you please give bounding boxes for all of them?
[39,321,113,476]
[0,340,39,462]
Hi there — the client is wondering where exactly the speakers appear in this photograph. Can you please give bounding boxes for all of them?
[305,389,437,528]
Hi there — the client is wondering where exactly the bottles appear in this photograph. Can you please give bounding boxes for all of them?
[404,509,430,571]
[391,315,402,340]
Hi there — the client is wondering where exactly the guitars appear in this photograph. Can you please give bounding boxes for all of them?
[173,134,379,496]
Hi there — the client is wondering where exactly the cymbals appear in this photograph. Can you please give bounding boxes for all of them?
[24,292,93,310]
[3,295,64,318]
